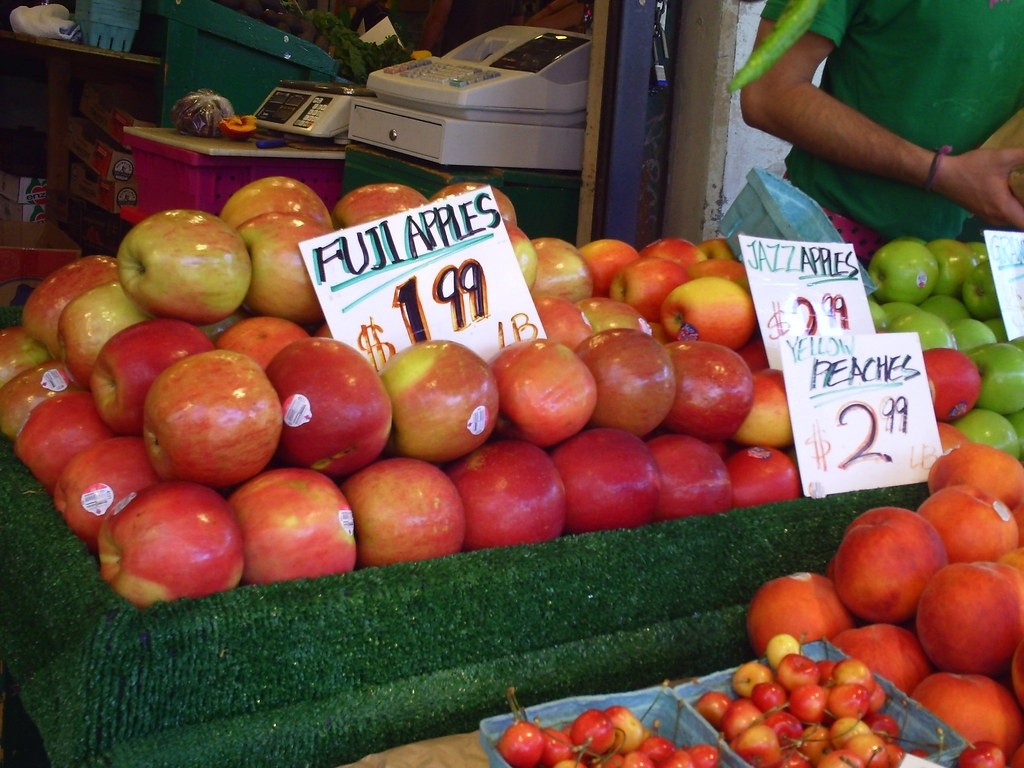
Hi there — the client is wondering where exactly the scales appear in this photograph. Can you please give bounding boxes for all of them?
[254,79,375,144]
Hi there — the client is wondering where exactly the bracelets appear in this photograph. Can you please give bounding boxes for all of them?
[546,3,556,14]
[920,145,953,192]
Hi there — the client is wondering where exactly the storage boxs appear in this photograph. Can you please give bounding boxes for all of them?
[75,0,142,52]
[0,67,346,308]
[480,638,967,768]
[718,165,877,298]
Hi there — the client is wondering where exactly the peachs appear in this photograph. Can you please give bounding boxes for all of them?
[747,443,1024,768]
[220,115,258,141]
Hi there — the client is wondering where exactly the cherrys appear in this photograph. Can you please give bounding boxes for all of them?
[498,633,1007,768]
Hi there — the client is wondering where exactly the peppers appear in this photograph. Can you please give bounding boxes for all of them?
[727,0,821,92]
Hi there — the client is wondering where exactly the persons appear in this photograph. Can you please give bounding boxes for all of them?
[418,0,593,56]
[740,0,1024,273]
[350,0,400,33]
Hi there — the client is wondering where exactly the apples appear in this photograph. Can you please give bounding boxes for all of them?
[867,237,1024,460]
[0,176,802,607]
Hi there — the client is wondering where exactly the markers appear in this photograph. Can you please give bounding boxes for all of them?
[253,139,285,148]
